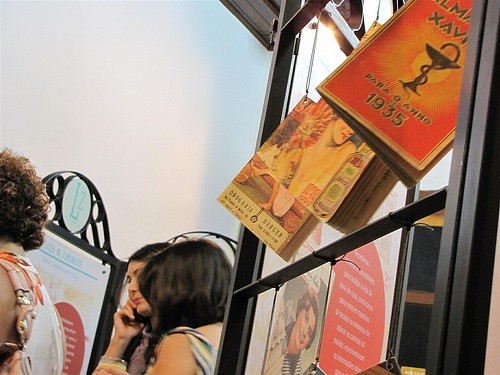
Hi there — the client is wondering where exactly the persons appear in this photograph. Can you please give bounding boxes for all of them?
[247,133,303,210]
[93,239,234,375]
[281,111,354,198]
[102,242,174,375]
[279,291,320,375]
[0,148,67,375]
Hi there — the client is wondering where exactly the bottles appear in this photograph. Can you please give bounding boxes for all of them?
[314,144,378,218]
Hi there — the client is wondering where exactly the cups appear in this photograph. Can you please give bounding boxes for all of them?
[98,354,128,375]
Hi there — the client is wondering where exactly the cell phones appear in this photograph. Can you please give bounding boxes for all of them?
[134,309,149,324]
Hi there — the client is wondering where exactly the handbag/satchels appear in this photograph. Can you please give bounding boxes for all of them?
[0,258,38,375]
